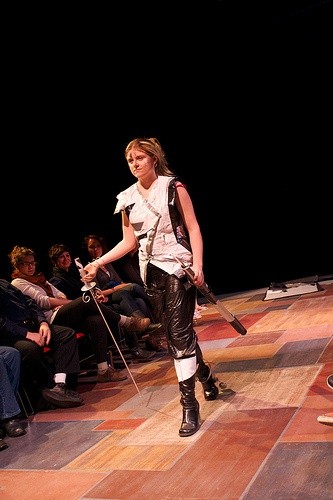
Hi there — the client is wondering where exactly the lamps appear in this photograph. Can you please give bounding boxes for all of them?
[269,282,287,292]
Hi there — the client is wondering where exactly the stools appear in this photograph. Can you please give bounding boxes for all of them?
[43,332,101,377]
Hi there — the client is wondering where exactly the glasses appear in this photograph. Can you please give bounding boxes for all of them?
[19,261,37,267]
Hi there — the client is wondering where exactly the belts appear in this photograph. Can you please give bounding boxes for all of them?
[19,319,34,328]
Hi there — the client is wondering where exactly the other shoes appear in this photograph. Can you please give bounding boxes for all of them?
[40,384,85,409]
[151,335,164,351]
[195,306,209,312]
[96,366,128,383]
[193,310,202,320]
[120,323,121,323]
[317,412,333,426]
[326,373,333,390]
[0,438,7,450]
[120,315,150,332]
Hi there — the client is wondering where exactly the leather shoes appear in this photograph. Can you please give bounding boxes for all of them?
[3,417,26,438]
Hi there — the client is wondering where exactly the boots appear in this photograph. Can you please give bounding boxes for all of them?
[167,333,202,438]
[130,346,157,364]
[196,342,220,401]
[130,309,162,342]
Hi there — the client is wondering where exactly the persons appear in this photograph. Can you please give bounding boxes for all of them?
[11,246,151,381]
[0,346,25,437]
[49,235,166,362]
[0,279,82,407]
[78,138,218,436]
